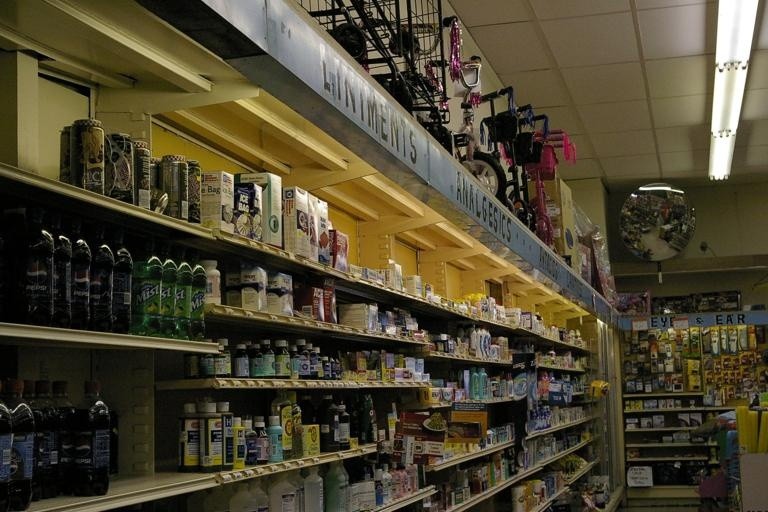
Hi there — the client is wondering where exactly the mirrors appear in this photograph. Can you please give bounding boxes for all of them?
[618,182,697,263]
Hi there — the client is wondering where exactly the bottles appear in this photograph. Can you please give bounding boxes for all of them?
[229,483,258,512]
[488,373,514,399]
[217,402,234,471]
[233,344,249,377]
[459,323,500,360]
[203,485,229,512]
[0,403,11,511]
[317,394,339,451]
[189,250,206,342]
[156,239,178,338]
[255,422,268,464]
[373,469,384,506]
[305,343,318,380]
[76,381,109,496]
[321,357,331,380]
[290,345,298,379]
[269,473,299,512]
[249,478,268,512]
[132,237,163,335]
[275,340,290,378]
[90,227,114,330]
[401,466,409,496]
[104,132,134,204]
[512,448,519,474]
[312,347,321,379]
[407,463,417,493]
[324,460,346,512]
[272,391,293,461]
[150,158,161,187]
[508,449,516,476]
[289,391,303,459]
[53,381,76,496]
[339,405,350,451]
[201,339,215,377]
[110,405,119,477]
[335,359,341,380]
[340,459,349,512]
[108,233,132,334]
[290,470,305,512]
[231,418,246,470]
[382,464,393,503]
[173,244,191,340]
[391,462,402,499]
[297,339,310,379]
[23,380,43,500]
[259,340,276,378]
[329,358,336,380]
[71,119,104,195]
[18,206,54,325]
[178,403,200,472]
[47,218,73,328]
[186,490,207,512]
[268,416,283,462]
[60,126,71,184]
[69,226,91,329]
[300,395,317,425]
[198,403,222,472]
[150,187,168,212]
[37,380,53,497]
[241,340,251,345]
[305,467,323,512]
[249,344,262,377]
[531,404,551,431]
[470,370,479,400]
[8,378,34,511]
[184,352,199,378]
[135,141,150,209]
[189,160,200,223]
[216,338,231,377]
[480,368,487,399]
[161,155,188,220]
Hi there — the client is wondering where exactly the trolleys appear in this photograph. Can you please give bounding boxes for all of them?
[304,0,452,155]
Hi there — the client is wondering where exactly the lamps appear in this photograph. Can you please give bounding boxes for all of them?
[709,0,760,182]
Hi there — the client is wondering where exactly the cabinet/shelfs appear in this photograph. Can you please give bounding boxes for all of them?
[0,0,625,512]
[618,289,768,507]
